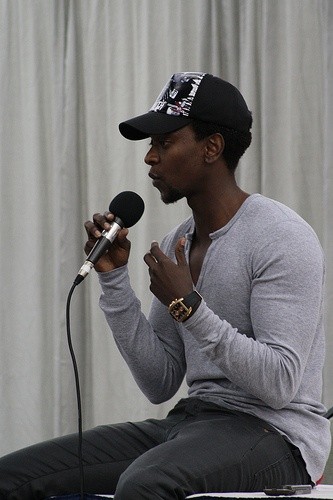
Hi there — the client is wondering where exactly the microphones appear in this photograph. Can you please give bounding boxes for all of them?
[74,191,146,286]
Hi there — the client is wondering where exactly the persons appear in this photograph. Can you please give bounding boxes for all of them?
[0,70,332,500]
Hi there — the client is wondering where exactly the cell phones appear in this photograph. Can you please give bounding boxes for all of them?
[266,488,293,496]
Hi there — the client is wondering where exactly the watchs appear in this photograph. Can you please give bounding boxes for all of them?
[166,290,203,323]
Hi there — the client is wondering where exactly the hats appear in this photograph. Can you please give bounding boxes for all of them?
[119,72,252,141]
